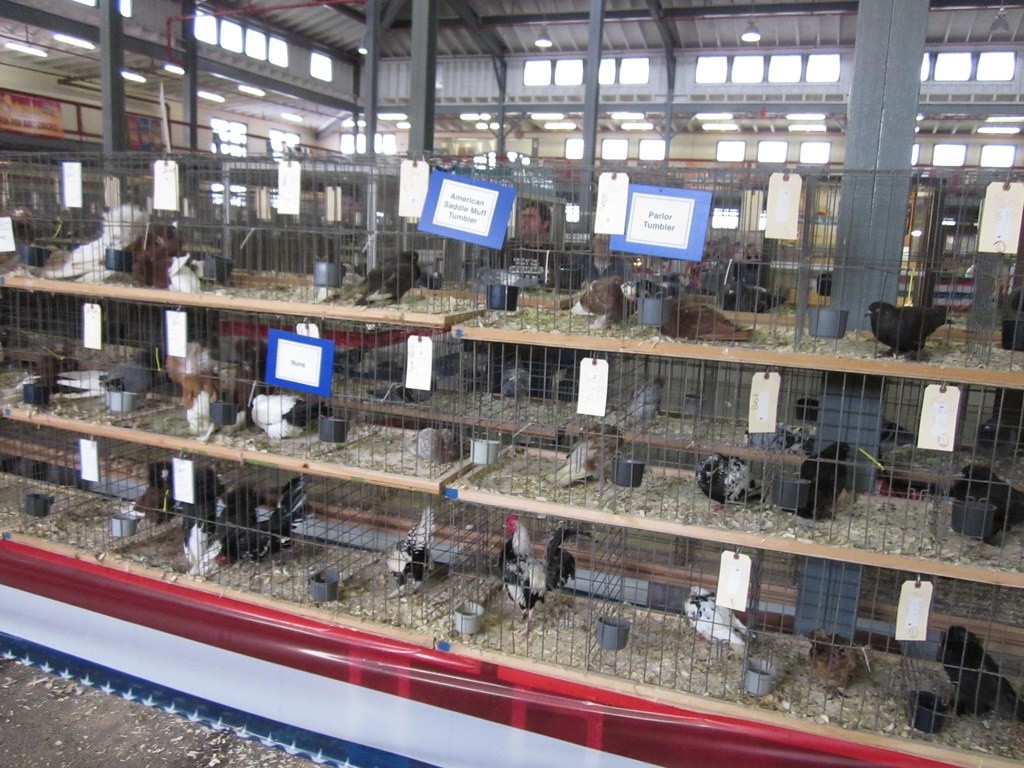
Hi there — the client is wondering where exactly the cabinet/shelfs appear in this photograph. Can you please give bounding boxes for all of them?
[1,147,1024,768]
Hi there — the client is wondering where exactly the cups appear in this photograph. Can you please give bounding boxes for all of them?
[13,242,1024,733]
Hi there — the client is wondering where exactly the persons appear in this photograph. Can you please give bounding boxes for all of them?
[990,284,1024,457]
[486,201,571,448]
[551,232,633,447]
[659,234,771,315]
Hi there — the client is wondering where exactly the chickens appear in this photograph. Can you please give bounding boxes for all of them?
[9,206,1024,722]
